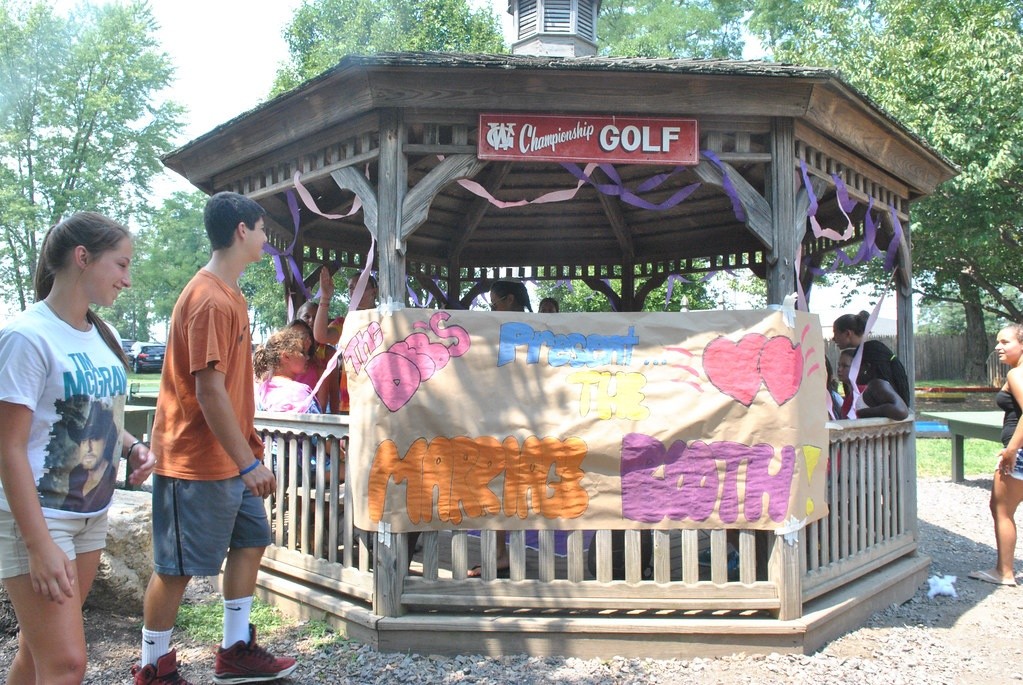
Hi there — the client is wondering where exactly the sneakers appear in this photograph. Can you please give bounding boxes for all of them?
[212,623,298,685]
[130,648,194,685]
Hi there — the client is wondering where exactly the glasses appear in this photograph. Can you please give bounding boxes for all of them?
[488,295,506,310]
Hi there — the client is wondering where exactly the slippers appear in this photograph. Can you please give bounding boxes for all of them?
[968,571,1016,586]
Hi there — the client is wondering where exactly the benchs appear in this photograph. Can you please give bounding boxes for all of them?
[274,478,375,569]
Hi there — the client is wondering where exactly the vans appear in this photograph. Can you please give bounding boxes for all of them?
[121,339,137,353]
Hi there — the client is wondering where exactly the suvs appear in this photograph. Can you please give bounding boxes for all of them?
[125,342,167,374]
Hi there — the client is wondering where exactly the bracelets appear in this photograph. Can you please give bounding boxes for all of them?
[126,440,143,461]
[238,458,260,476]
[319,302,329,307]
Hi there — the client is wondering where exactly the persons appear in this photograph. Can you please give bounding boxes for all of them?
[132,192,302,685]
[824,309,910,562]
[312,266,422,571]
[538,297,560,313]
[466,277,534,578]
[0,212,157,685]
[965,322,1023,586]
[253,301,345,556]
[699,529,767,576]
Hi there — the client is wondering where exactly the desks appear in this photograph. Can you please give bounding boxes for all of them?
[922,410,1008,488]
